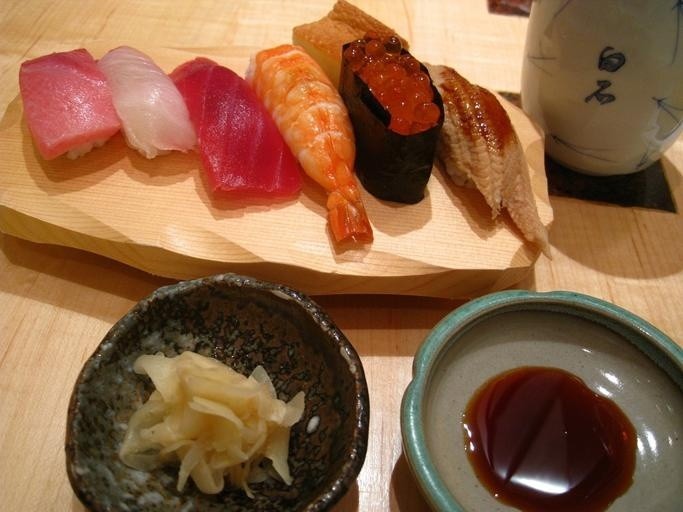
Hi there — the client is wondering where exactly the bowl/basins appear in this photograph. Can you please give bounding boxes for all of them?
[64,270,368,512]
[400,290,682,512]
